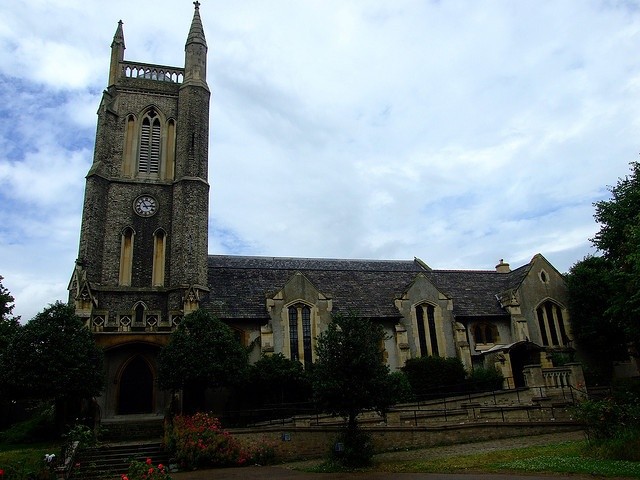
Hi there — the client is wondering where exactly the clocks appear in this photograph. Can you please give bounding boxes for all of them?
[133,194,159,218]
